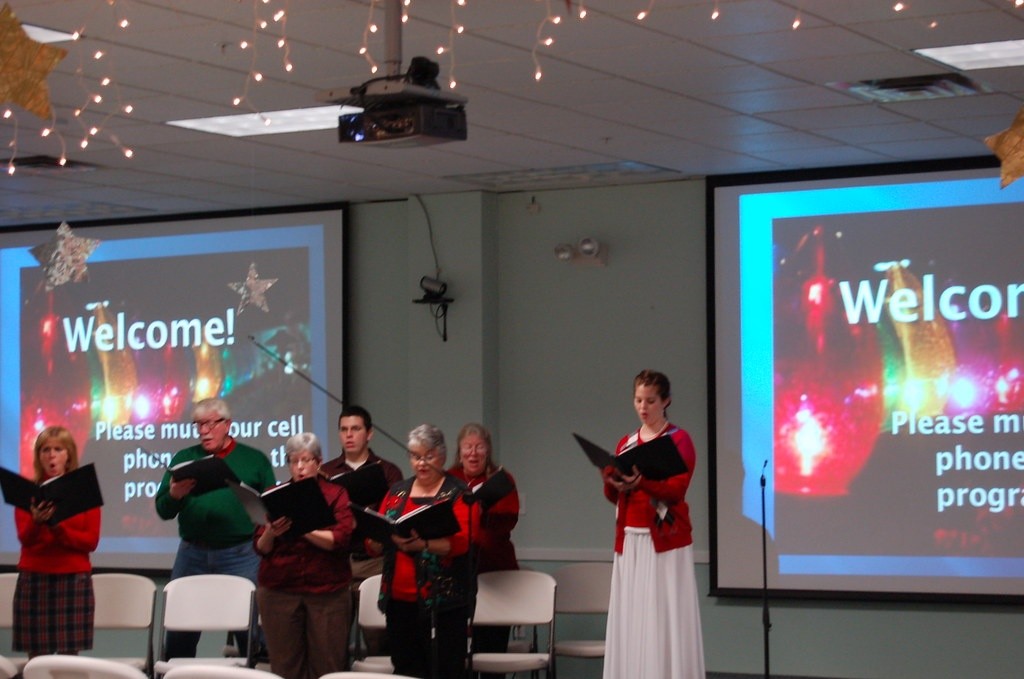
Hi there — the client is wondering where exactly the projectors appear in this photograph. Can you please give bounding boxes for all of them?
[342,104,467,148]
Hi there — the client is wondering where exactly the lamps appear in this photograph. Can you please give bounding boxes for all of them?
[553,236,609,268]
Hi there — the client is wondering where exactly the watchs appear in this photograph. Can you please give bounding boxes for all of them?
[423,540,429,551]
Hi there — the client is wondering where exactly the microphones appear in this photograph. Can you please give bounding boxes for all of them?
[761,460,767,479]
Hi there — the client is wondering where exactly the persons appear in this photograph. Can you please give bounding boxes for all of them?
[567,368,706,679]
[378,423,477,679]
[447,423,520,678]
[12,425,102,658]
[155,396,276,661]
[253,431,356,675]
[322,404,403,540]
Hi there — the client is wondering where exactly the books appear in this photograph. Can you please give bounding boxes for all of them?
[363,497,461,538]
[0,462,103,521]
[137,447,241,498]
[468,465,515,509]
[238,477,341,536]
[570,433,690,480]
[327,459,391,503]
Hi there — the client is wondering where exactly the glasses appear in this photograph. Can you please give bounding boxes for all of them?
[290,456,315,466]
[192,416,225,430]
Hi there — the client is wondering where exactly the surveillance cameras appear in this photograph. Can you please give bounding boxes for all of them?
[420,275,447,294]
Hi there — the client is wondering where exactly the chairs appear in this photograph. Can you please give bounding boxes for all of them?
[0,562,614,679]
